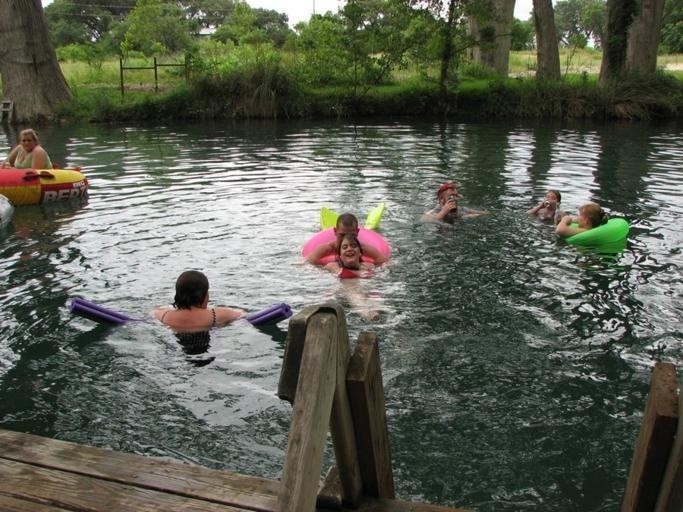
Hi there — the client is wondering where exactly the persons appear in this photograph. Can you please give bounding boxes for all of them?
[151,271,244,352]
[306,214,388,265]
[421,183,491,223]
[326,234,380,322]
[555,204,607,237]
[8,129,52,170]
[527,188,566,223]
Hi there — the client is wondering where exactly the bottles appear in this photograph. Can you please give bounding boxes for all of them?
[568,216,579,224]
[449,196,458,217]
[544,201,551,207]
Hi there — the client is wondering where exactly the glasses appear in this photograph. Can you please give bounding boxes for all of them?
[446,193,466,200]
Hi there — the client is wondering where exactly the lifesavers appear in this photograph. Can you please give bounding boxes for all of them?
[301,225,391,264]
[554,217,629,246]
[1,165,88,207]
